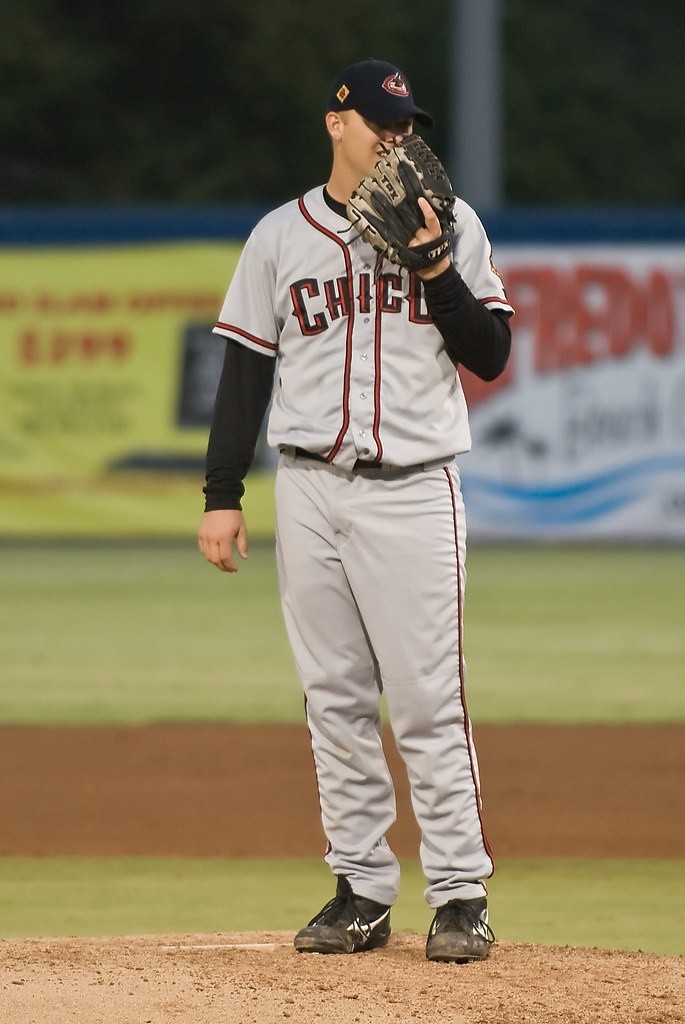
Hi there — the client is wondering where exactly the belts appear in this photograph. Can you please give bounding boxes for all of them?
[279,445,382,470]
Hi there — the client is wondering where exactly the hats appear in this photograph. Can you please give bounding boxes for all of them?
[330,59,434,130]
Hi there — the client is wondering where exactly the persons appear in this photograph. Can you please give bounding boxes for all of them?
[197,57,514,962]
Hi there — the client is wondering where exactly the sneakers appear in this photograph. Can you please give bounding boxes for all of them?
[293,873,391,954]
[425,898,495,962]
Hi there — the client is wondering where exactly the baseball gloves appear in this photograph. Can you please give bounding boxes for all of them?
[343,133,461,274]
